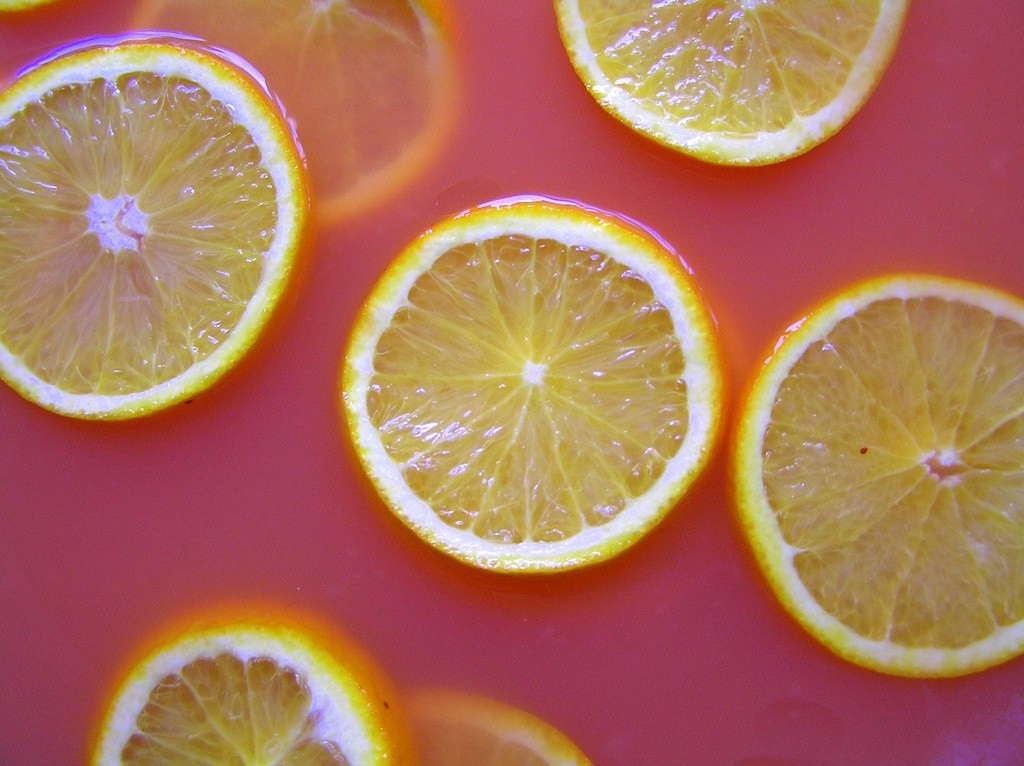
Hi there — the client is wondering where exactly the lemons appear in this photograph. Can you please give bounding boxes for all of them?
[1,44,306,421]
[729,274,1024,678]
[341,203,723,575]
[552,0,908,167]
[122,1,460,226]
[401,690,591,766]
[91,607,399,766]
